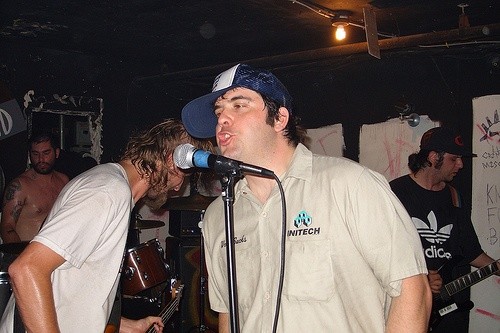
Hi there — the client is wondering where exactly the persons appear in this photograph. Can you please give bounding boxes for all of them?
[182,63,432,333]
[0,131,70,244]
[0,118,216,333]
[387,126,500,333]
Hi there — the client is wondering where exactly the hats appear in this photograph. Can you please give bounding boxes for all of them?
[181,62,296,139]
[420,127,477,157]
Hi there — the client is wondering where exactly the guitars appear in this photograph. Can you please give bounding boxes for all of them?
[104,283,183,333]
[429,258,500,331]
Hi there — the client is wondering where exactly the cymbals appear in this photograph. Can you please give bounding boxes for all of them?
[124,221,164,229]
[0,240,29,255]
[164,196,218,211]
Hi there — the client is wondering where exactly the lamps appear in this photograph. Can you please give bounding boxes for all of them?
[457,3,470,31]
[399,104,420,128]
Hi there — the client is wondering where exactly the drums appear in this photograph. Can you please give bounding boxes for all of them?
[124,239,177,297]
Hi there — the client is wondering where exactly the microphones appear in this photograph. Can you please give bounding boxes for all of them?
[400,112,420,127]
[173,143,274,176]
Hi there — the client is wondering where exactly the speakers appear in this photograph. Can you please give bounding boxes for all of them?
[166,235,219,333]
